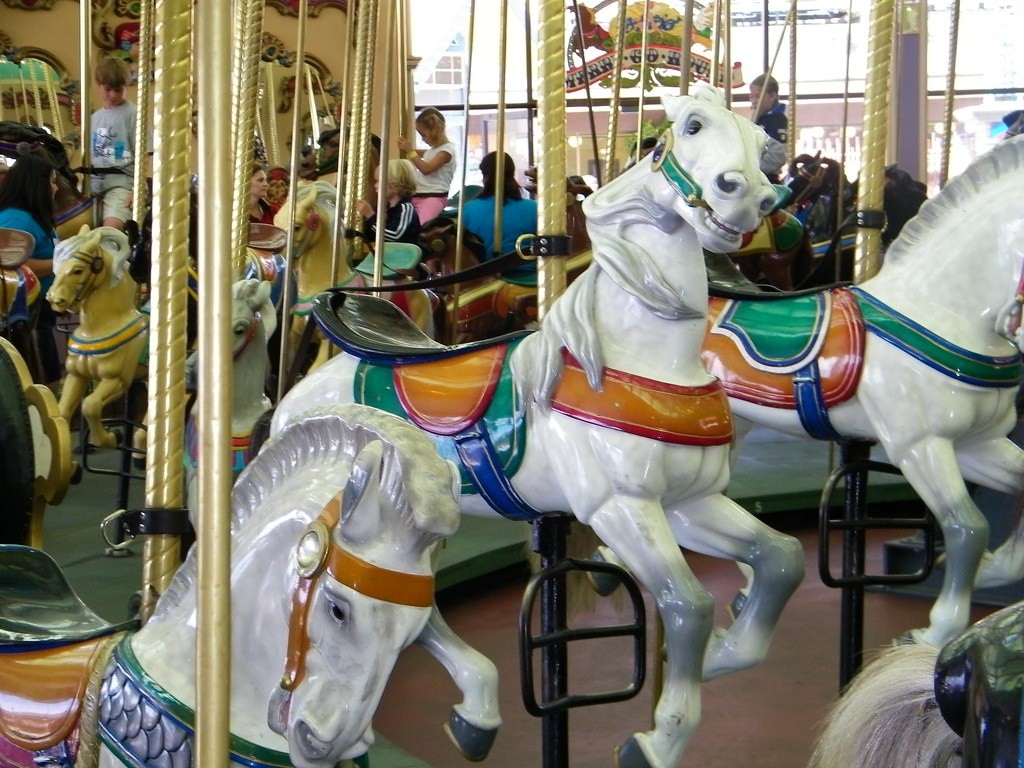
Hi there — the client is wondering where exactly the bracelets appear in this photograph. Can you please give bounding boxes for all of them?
[405,150,418,159]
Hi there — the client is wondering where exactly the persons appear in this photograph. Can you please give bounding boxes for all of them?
[749,74,784,181]
[249,160,281,225]
[355,158,424,246]
[77,57,137,232]
[397,107,457,225]
[0,154,62,397]
[317,128,347,175]
[457,150,538,271]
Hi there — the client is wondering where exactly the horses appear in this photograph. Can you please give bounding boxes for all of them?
[242,79,810,768]
[697,129,1024,655]
[43,174,438,544]
[0,402,464,768]
